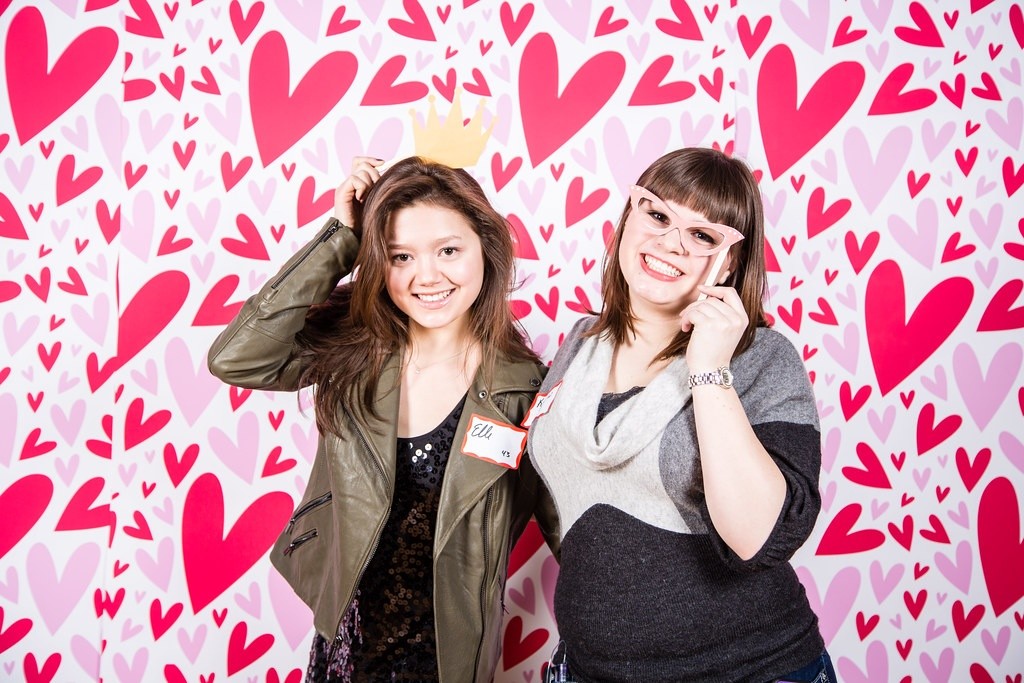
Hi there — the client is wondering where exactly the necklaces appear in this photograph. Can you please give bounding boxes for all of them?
[404,346,441,374]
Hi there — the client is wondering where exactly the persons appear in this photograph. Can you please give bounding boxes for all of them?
[526,148,836,683]
[209,156,551,683]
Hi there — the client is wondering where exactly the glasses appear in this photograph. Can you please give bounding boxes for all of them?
[630,184,745,257]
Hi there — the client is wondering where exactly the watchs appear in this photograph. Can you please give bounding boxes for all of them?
[689,367,735,388]
